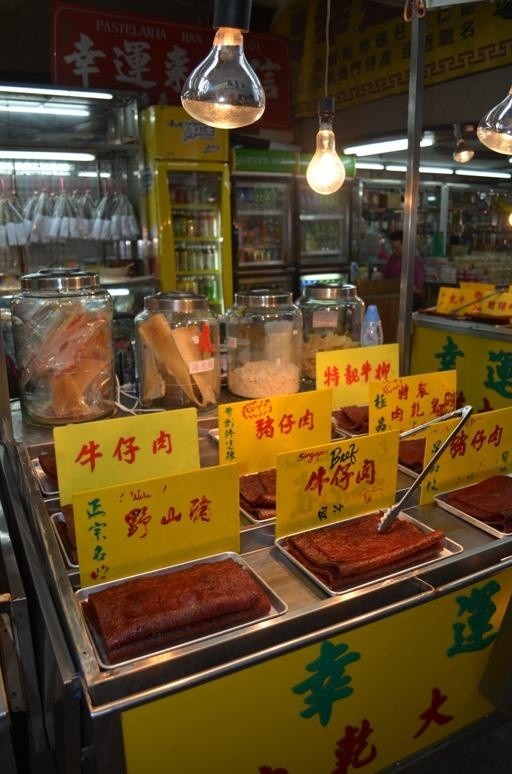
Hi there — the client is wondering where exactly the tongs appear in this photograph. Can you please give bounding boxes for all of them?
[0,190,141,248]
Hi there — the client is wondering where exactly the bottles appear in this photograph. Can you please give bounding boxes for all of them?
[237,218,282,244]
[225,289,303,399]
[135,291,222,419]
[175,244,218,271]
[173,210,218,237]
[169,184,210,204]
[9,266,116,430]
[361,305,384,348]
[294,282,366,386]
[176,275,218,300]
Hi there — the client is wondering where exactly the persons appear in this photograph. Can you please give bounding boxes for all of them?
[383,230,424,311]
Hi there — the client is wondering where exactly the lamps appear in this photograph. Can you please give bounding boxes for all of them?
[0,85,115,100]
[0,150,112,179]
[342,129,439,157]
[0,104,91,117]
